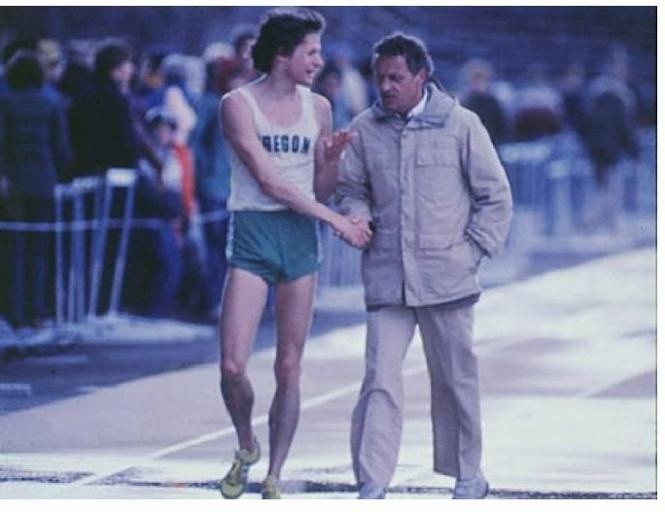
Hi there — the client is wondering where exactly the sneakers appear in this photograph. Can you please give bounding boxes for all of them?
[220,437,260,498]
[261,473,282,499]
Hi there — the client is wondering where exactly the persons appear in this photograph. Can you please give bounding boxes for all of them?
[330,30,516,499]
[460,51,656,232]
[318,47,373,130]
[2,26,257,332]
[217,7,377,499]
